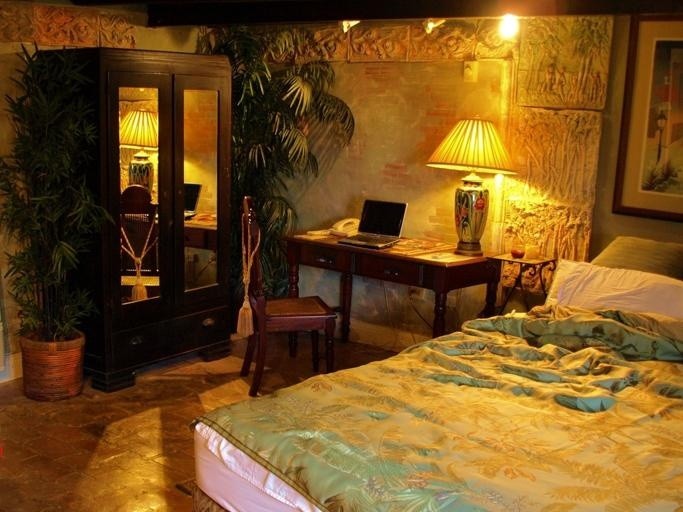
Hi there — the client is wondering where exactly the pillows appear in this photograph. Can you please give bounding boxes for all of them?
[545,235,682,330]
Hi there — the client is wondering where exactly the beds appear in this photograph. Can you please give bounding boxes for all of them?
[188,306,683,511]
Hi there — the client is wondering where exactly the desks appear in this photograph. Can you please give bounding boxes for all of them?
[486,253,557,315]
[278,232,500,363]
[124,212,218,277]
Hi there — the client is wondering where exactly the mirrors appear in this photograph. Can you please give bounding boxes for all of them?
[115,86,163,307]
[182,89,224,292]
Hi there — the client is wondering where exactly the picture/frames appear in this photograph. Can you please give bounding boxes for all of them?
[613,12,682,226]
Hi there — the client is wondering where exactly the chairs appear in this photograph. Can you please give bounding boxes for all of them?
[237,196,336,400]
[117,186,157,297]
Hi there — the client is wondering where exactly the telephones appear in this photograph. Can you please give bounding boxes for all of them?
[329,218,361,237]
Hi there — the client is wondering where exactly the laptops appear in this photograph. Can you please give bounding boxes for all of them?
[337,199,408,250]
[147,183,202,220]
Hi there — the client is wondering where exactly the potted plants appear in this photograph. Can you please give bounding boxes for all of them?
[1,46,116,400]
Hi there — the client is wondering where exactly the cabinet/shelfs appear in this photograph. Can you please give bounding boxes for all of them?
[37,47,234,393]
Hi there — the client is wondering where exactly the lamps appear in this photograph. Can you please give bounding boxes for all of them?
[425,119,519,257]
[117,107,158,195]
